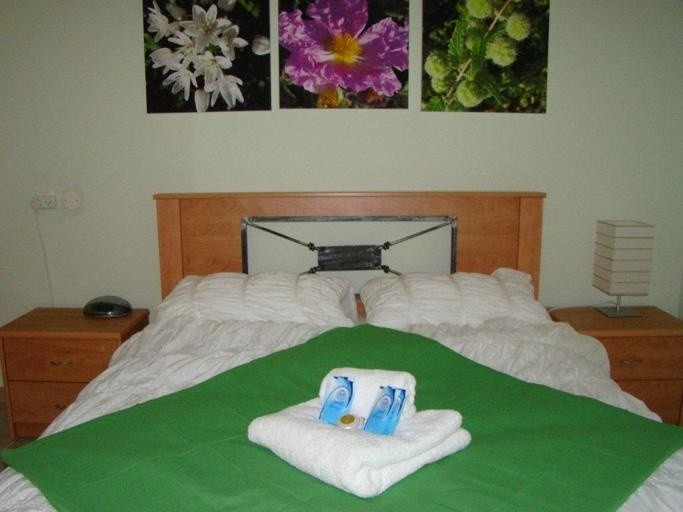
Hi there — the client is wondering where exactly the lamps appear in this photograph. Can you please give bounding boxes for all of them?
[592,220,655,317]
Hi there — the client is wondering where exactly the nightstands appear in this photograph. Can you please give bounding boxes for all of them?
[0,307,150,440]
[547,306,683,425]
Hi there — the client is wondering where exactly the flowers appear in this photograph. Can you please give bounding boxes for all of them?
[278,0,409,108]
[147,0,271,113]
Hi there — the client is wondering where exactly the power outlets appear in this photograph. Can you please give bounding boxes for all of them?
[31,196,56,210]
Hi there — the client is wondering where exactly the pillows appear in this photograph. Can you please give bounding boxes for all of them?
[360,272,552,328]
[153,272,357,326]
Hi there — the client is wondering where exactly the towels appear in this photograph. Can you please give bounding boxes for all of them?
[247,397,472,499]
[319,367,417,421]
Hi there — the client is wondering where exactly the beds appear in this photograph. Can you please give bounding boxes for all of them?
[0,192,683,512]
